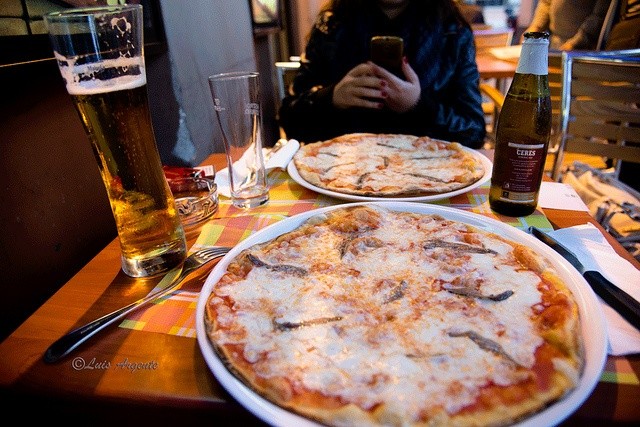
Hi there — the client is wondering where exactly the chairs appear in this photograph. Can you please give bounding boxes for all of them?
[469,29,515,136]
[550,49,640,203]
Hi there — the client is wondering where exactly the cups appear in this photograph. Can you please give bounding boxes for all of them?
[208,70,270,206]
[45,4,188,279]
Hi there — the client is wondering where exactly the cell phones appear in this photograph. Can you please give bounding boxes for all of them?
[371,35,404,80]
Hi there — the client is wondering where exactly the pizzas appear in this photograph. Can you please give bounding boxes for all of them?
[293,132,486,195]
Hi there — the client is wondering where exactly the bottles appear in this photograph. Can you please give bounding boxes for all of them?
[489,32,552,216]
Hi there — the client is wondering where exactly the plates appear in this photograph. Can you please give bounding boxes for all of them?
[287,133,494,201]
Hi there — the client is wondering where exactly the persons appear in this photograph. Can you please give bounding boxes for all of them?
[275,0,486,149]
[518,0,608,50]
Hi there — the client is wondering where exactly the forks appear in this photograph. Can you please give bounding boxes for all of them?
[42,247,229,364]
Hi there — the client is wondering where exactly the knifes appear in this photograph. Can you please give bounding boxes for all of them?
[528,225,637,320]
